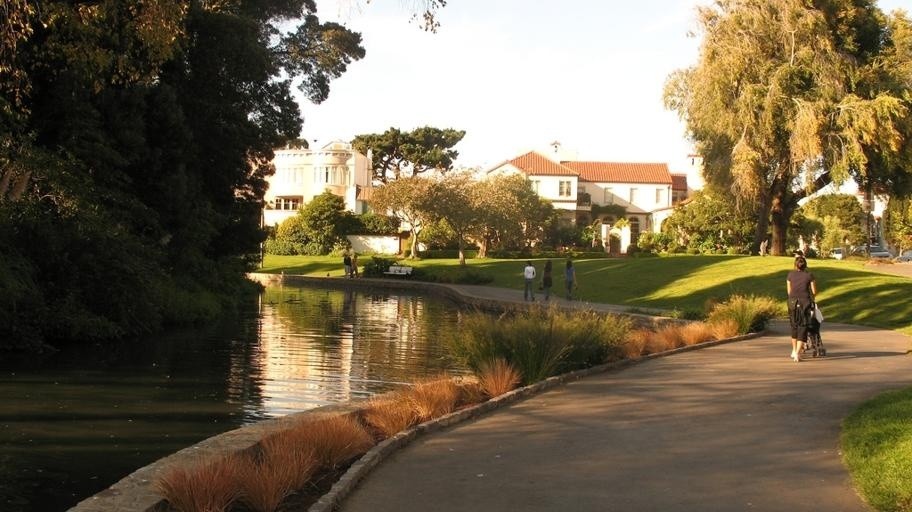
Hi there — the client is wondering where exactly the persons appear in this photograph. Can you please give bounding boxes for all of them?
[344,253,352,276]
[543,261,552,300]
[524,262,536,301]
[786,257,816,362]
[565,260,577,300]
[350,255,358,277]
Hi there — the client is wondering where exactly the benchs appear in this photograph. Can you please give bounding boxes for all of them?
[383,266,413,279]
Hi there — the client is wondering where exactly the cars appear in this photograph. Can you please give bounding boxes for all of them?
[851,245,889,257]
[828,247,842,260]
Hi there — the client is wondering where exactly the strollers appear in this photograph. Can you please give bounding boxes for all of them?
[800,294,825,357]
